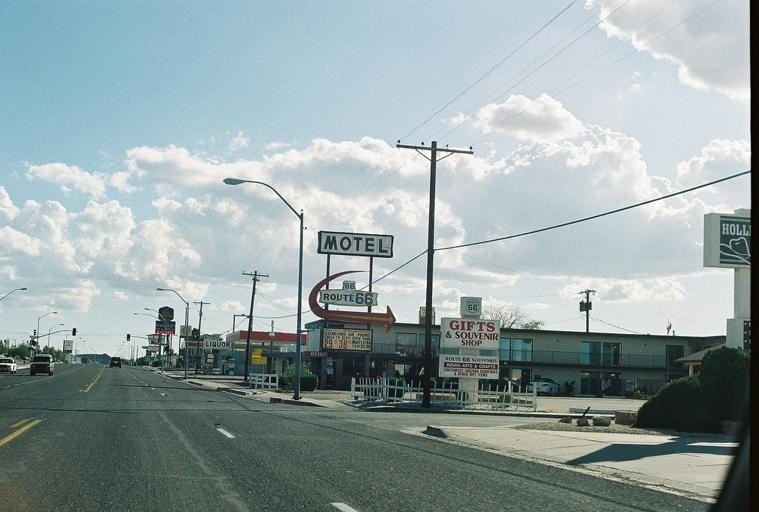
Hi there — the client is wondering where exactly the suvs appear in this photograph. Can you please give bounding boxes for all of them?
[28,353,55,376]
[109,357,122,369]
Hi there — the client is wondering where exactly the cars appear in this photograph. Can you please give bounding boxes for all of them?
[486,377,520,392]
[0,358,17,374]
[525,378,560,396]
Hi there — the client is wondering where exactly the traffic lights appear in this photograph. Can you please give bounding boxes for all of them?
[127,334,130,341]
[72,328,75,336]
[33,329,36,336]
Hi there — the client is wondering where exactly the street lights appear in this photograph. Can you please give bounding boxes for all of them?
[36,311,58,354]
[223,177,306,402]
[157,288,190,380]
[48,323,65,348]
[0,287,26,301]
[133,312,159,319]
[117,340,135,366]
[266,320,275,374]
[144,308,166,373]
